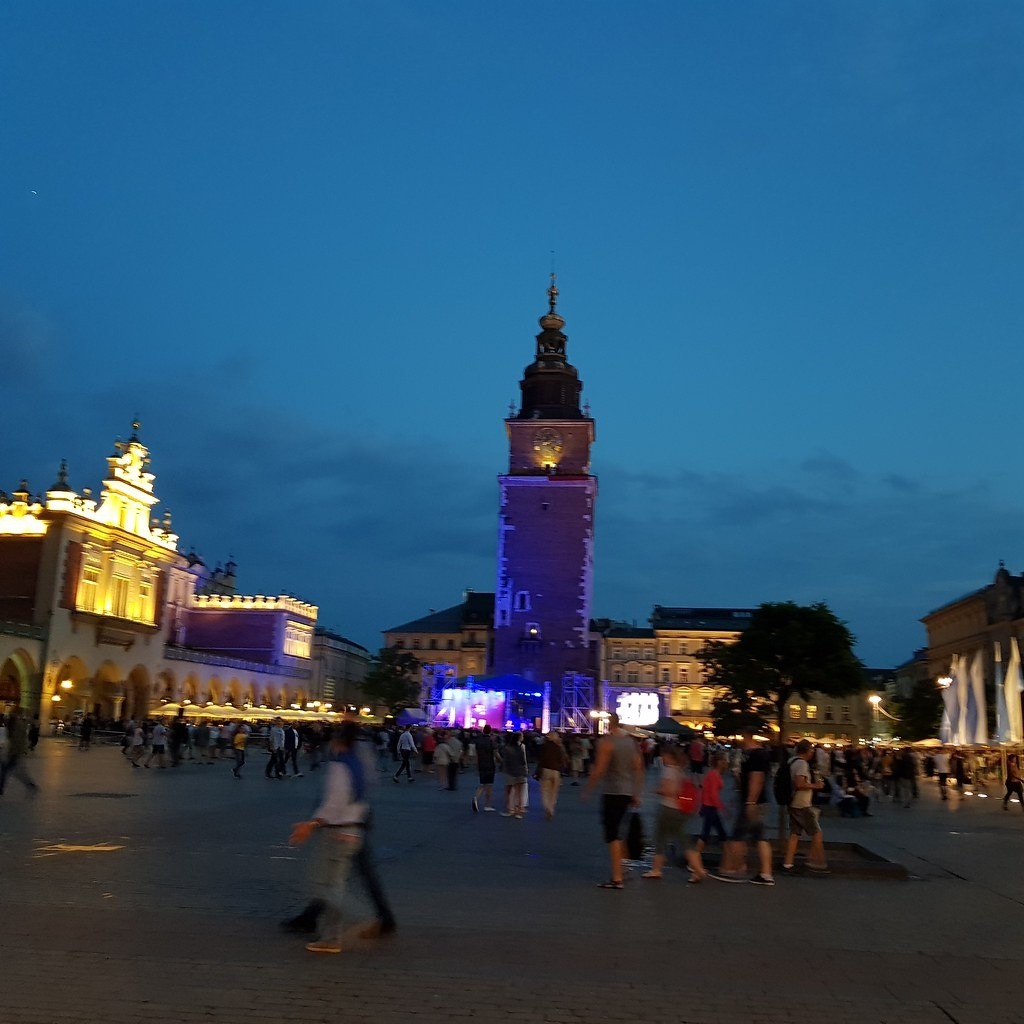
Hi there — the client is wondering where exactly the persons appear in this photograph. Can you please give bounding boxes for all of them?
[472,725,500,813]
[0,705,1024,952]
[538,730,567,821]
[392,724,419,783]
[1002,754,1024,810]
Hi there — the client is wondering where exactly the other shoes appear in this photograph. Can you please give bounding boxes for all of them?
[516,812,524,819]
[408,778,416,782]
[121,754,306,780]
[861,783,1017,818]
[500,810,513,816]
[805,862,833,874]
[281,916,321,941]
[709,871,748,883]
[750,874,776,886]
[392,777,400,783]
[471,796,479,814]
[306,937,343,954]
[781,864,804,875]
[484,808,497,812]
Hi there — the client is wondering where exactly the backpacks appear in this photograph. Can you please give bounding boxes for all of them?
[670,766,699,814]
[773,758,808,804]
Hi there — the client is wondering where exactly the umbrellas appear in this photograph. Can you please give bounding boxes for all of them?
[151,700,384,725]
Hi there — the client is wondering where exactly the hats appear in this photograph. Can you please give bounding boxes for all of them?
[483,725,491,735]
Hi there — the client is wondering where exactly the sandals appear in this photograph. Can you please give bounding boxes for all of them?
[641,869,662,878]
[688,870,707,882]
[597,878,624,889]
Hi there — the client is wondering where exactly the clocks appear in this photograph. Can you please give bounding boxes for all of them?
[532,428,566,462]
